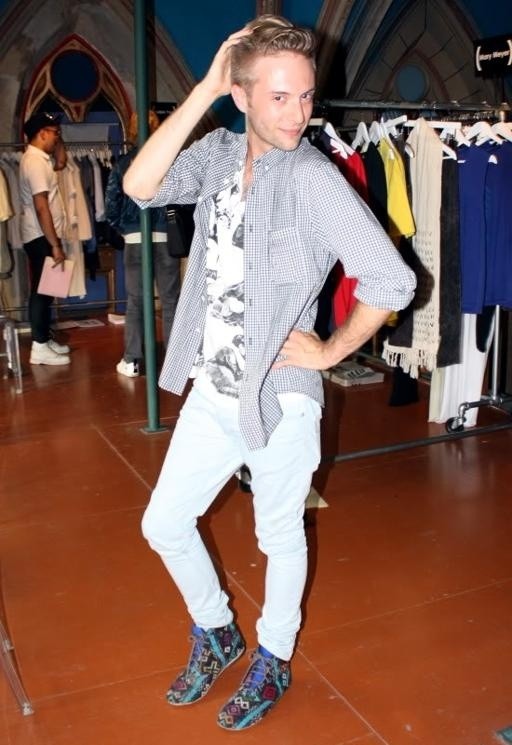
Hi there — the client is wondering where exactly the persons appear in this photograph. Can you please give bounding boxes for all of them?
[20,112,73,365]
[106,108,195,377]
[122,17,416,731]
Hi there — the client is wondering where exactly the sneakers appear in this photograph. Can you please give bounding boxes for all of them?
[115,358,141,379]
[166,619,248,706]
[214,641,295,732]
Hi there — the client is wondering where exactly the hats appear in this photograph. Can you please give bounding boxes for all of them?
[21,110,62,144]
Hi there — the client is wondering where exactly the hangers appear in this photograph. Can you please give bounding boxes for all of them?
[320,100,511,167]
[2,139,136,169]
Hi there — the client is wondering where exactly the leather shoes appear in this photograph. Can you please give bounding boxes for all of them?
[28,341,71,367]
[46,339,71,354]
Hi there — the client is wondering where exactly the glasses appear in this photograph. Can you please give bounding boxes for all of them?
[41,128,62,137]
